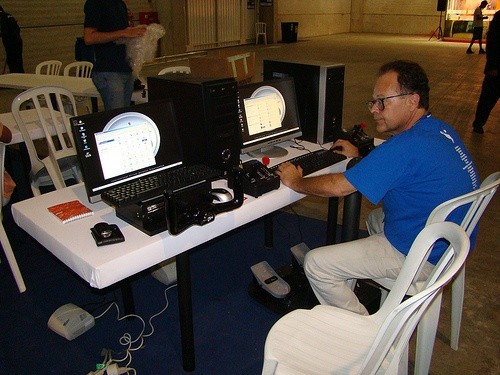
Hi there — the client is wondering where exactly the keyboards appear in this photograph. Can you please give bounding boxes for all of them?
[263,58,345,145]
[267,149,347,179]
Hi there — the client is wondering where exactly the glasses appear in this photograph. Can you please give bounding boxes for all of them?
[367,93,413,111]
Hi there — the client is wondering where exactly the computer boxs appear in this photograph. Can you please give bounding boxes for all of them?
[146,74,242,181]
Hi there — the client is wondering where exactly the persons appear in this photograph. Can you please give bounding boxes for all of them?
[274,59,481,317]
[0,5,24,73]
[466,0,500,132]
[83,0,146,112]
[0,123,15,205]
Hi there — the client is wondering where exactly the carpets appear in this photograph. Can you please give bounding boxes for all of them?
[0,146,380,375]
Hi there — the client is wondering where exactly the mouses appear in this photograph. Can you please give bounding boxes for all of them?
[332,146,343,151]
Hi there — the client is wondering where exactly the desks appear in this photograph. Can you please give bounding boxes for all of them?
[12,139,354,375]
[0,73,147,113]
[0,107,74,205]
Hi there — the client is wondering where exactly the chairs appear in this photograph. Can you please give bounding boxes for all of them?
[262,222,470,375]
[369,167,500,375]
[0,53,253,293]
[255,22,267,45]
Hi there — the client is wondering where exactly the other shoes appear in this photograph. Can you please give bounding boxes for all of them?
[479,49,486,53]
[466,49,474,54]
[473,122,483,133]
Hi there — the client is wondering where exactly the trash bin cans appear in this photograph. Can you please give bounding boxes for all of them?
[281,22,298,42]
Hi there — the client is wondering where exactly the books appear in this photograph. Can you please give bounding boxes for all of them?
[48,199,94,225]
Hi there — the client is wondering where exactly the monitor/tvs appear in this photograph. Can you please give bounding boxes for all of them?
[69,97,185,204]
[237,76,302,159]
